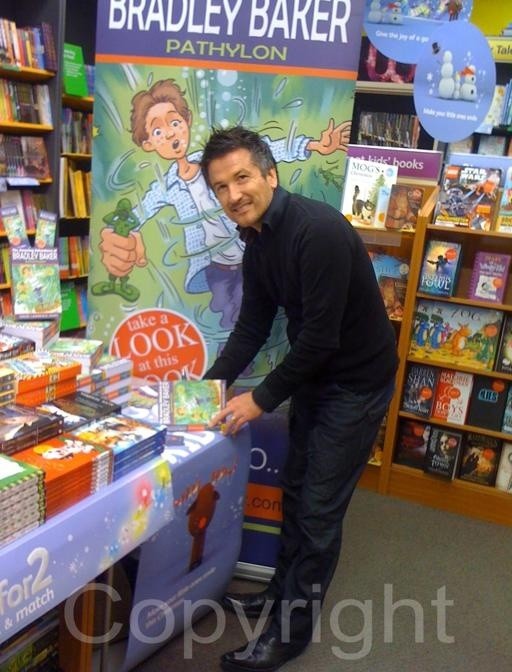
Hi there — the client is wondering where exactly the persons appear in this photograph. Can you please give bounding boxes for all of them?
[197,123,402,672]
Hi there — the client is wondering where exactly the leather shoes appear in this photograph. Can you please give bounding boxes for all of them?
[221,589,276,617]
[219,630,312,671]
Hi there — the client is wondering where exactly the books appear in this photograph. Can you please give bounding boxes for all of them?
[340,137,512,496]
[3,18,236,547]
